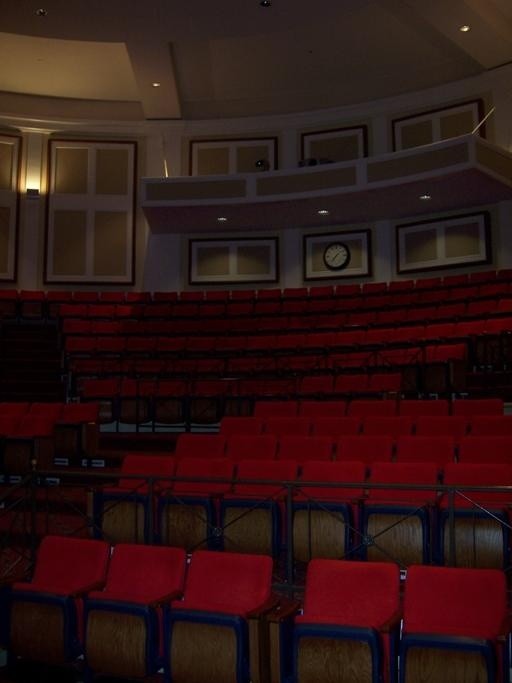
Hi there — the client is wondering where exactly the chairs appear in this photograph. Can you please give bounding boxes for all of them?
[1,290,280,437]
[1,437,511,683]
[280,269,511,437]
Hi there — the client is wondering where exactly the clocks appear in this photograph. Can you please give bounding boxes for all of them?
[322,242,351,272]
